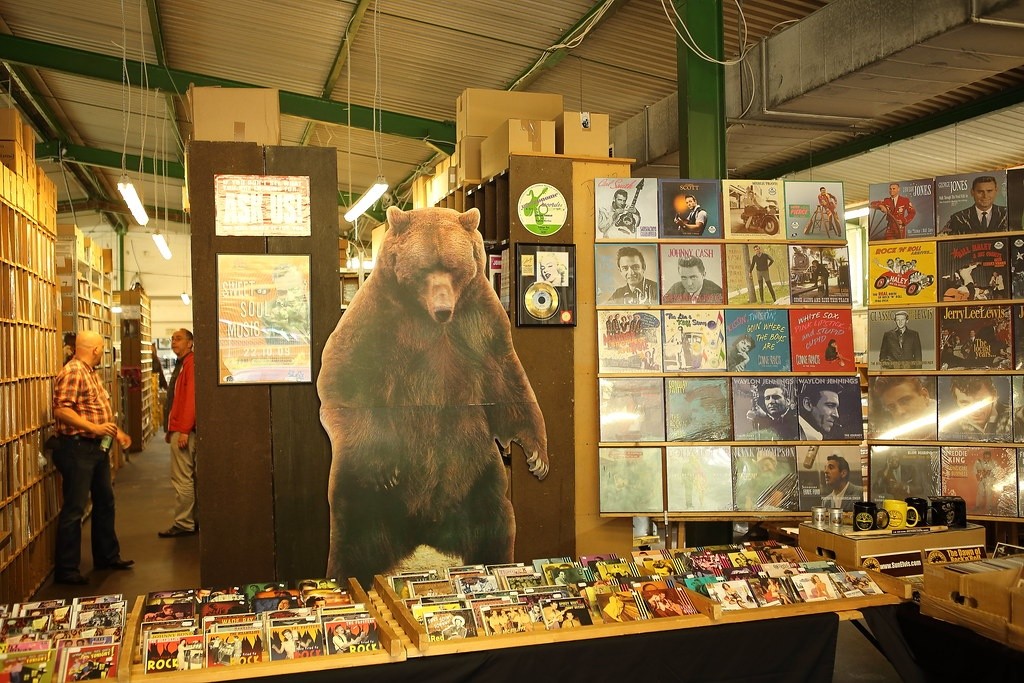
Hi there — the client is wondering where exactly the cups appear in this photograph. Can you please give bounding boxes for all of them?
[882,499,918,529]
[905,497,939,526]
[852,502,890,531]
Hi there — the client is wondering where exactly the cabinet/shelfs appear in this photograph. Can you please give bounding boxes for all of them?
[0,197,167,606]
[436,153,641,563]
[188,141,342,589]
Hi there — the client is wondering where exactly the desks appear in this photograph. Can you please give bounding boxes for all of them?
[0,559,911,683]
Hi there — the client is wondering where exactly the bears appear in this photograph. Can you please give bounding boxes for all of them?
[315,206,551,591]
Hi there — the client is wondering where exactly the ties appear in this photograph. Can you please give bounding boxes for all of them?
[981,213,988,232]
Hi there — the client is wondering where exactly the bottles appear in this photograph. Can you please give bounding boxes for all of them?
[811,506,827,527]
[828,508,844,527]
[99,412,119,453]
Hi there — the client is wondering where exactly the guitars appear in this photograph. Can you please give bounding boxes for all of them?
[750,383,761,440]
[676,212,690,233]
[607,178,645,239]
[548,606,573,627]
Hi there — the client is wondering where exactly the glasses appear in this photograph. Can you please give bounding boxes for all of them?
[170,337,190,342]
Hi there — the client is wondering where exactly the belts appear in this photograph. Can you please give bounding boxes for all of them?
[59,434,101,442]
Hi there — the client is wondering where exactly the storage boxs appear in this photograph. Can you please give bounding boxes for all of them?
[186,82,281,146]
[0,107,113,272]
[371,87,608,266]
[799,522,1024,652]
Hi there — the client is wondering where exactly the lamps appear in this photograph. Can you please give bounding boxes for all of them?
[344,0,389,223]
[103,0,189,305]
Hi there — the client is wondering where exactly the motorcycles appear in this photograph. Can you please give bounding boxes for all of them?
[741,206,779,236]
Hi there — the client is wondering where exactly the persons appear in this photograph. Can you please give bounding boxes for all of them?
[793,247,829,297]
[663,256,723,303]
[938,176,1007,235]
[958,262,1005,301]
[747,379,845,441]
[606,313,653,368]
[600,446,1017,516]
[826,340,852,367]
[62,332,92,525]
[157,328,197,537]
[0,538,884,683]
[727,334,756,372]
[939,317,1009,370]
[869,183,916,239]
[879,311,922,369]
[52,331,135,582]
[818,187,840,230]
[599,188,641,238]
[539,253,567,285]
[674,197,709,236]
[607,247,657,304]
[873,375,1013,442]
[749,245,777,302]
[743,191,763,231]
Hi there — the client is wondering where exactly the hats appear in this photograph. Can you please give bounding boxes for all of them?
[757,451,777,466]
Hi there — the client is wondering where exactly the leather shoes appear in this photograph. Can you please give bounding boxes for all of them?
[54,563,89,584]
[94,554,134,569]
[159,526,194,537]
